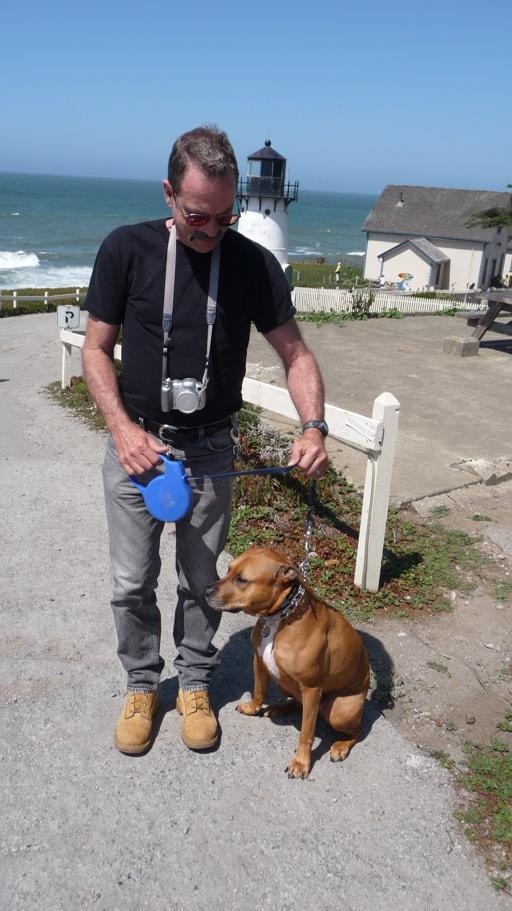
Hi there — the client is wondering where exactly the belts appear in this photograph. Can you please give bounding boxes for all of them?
[132,414,238,443]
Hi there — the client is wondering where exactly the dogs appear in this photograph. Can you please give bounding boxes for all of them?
[204,541,371,780]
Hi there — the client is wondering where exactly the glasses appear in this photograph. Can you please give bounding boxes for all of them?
[174,192,240,226]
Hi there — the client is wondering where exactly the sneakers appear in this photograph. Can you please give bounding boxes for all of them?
[177,688,219,749]
[117,692,158,754]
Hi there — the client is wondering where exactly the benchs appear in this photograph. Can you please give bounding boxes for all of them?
[467,308,512,331]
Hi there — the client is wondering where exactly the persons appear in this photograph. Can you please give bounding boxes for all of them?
[82,126,328,754]
[334,262,341,284]
[469,283,475,289]
[503,275,511,289]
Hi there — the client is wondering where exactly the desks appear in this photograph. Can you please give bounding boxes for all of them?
[471,288,512,341]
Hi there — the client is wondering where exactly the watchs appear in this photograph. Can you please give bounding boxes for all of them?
[303,420,328,439]
